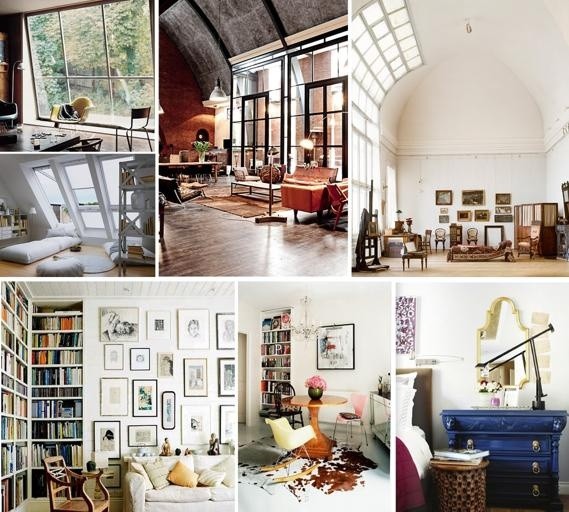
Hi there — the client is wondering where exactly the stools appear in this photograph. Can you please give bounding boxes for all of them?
[401,251,428,272]
[34,259,84,277]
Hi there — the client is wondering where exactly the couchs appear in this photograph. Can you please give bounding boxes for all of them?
[120,456,238,512]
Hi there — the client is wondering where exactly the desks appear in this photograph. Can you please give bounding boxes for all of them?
[428,459,490,512]
[278,394,348,461]
[382,234,418,260]
[0,127,81,153]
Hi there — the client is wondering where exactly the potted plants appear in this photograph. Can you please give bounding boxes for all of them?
[191,139,215,162]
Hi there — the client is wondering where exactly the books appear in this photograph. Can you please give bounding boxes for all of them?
[261,313,290,420]
[2,281,84,511]
[434,450,490,459]
[0,214,30,239]
[120,171,155,260]
[432,455,483,465]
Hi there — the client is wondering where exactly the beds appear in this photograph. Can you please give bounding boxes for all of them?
[0,231,84,265]
[396,367,441,512]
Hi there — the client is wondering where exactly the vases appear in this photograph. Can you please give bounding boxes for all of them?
[307,388,324,401]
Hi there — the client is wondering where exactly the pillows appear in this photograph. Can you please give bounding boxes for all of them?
[395,388,418,438]
[47,228,65,238]
[58,223,77,238]
[397,371,421,390]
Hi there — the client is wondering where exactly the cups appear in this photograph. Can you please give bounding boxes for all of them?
[490,398,500,407]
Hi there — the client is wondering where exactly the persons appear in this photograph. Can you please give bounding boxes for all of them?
[158,438,174,456]
[207,433,220,455]
[187,319,201,342]
[224,320,235,344]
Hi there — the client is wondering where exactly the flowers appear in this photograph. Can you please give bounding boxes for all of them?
[303,375,328,390]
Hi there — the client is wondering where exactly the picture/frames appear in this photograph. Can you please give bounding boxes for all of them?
[367,221,378,236]
[432,188,513,224]
[316,322,357,373]
[93,305,236,491]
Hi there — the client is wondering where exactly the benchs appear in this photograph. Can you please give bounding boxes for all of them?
[281,165,338,186]
[280,182,348,225]
[446,238,514,263]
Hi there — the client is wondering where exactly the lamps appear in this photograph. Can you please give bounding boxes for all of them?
[10,60,27,129]
[480,350,527,381]
[255,146,288,224]
[396,208,405,222]
[299,109,348,151]
[208,0,228,103]
[28,207,38,242]
[291,296,322,342]
[463,15,474,36]
[475,323,556,411]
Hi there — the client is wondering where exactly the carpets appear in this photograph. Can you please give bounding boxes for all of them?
[238,425,380,505]
[186,184,293,218]
[55,255,117,275]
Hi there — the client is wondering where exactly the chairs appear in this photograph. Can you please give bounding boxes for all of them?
[159,175,215,211]
[233,166,261,189]
[159,161,224,183]
[40,456,112,512]
[273,381,305,432]
[333,392,372,448]
[516,218,543,263]
[256,416,322,484]
[418,230,433,255]
[433,228,445,252]
[325,183,348,231]
[466,228,478,247]
[0,101,19,126]
[49,97,95,137]
[115,106,153,154]
[67,138,104,152]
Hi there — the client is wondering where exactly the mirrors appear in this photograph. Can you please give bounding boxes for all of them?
[477,295,531,391]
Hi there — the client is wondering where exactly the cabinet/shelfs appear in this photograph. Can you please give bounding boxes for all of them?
[449,223,463,248]
[0,213,30,245]
[0,282,33,512]
[440,409,568,512]
[259,305,301,414]
[29,297,88,499]
[370,391,391,453]
[179,149,228,175]
[117,159,156,278]
